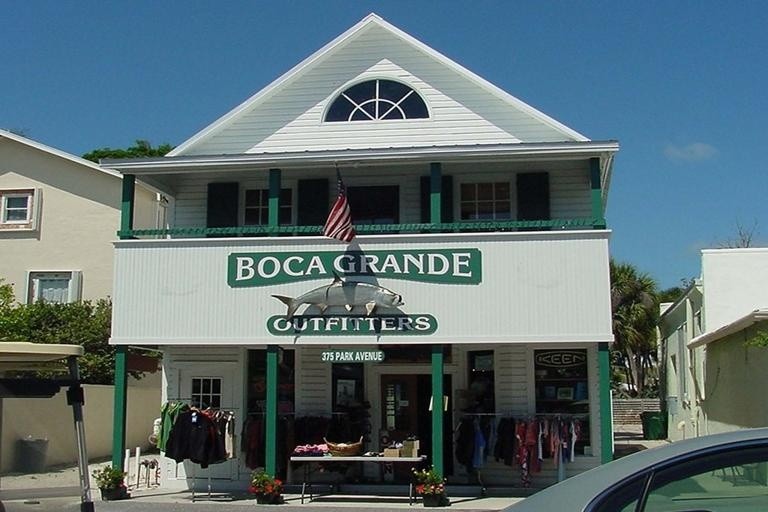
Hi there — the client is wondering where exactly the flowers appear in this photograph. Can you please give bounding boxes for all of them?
[248,470,284,496]
[93,466,129,486]
[410,462,448,495]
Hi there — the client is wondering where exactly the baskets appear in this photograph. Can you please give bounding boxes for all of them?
[323,434,365,457]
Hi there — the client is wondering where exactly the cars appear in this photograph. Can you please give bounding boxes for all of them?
[611,380,637,399]
[497,426,766,512]
[551,397,600,449]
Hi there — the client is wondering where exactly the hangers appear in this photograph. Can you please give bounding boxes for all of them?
[164,398,236,422]
[459,411,579,426]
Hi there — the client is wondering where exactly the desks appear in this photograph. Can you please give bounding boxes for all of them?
[291,454,428,505]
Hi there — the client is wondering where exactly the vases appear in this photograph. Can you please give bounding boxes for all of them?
[421,492,442,507]
[255,490,281,504]
[100,485,127,500]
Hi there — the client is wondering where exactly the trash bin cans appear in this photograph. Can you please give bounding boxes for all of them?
[20,438,49,474]
[639,411,667,440]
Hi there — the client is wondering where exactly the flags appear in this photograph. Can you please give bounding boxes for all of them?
[320,166,356,242]
